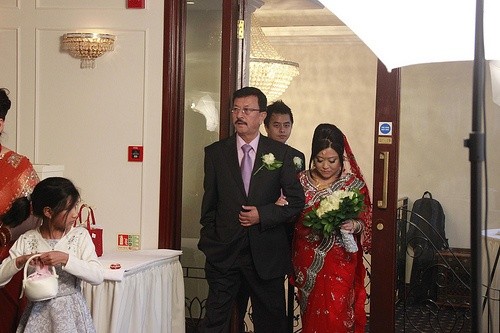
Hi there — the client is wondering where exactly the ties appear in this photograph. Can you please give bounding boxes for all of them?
[241,144,255,195]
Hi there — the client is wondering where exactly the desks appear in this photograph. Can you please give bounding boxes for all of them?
[80,249,186,333]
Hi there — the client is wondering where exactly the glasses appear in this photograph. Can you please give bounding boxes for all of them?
[231,106,260,115]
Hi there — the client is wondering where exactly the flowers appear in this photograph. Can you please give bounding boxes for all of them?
[303,188,365,253]
[252,153,284,176]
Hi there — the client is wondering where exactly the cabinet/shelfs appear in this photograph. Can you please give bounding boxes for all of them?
[434,246,471,307]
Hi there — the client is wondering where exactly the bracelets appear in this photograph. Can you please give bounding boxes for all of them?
[0,226,13,244]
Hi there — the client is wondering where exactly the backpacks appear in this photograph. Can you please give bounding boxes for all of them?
[406,190,449,256]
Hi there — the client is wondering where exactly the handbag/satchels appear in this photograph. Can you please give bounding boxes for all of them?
[73,204,104,259]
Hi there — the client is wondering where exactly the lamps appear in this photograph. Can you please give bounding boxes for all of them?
[248,15,299,105]
[62,33,115,69]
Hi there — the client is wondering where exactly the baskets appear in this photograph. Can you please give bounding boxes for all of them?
[19,254,60,304]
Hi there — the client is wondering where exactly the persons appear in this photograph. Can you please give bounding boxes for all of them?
[266,101,306,173]
[0,88,40,333]
[197,87,305,332]
[275,124,373,333]
[0,177,104,333]
